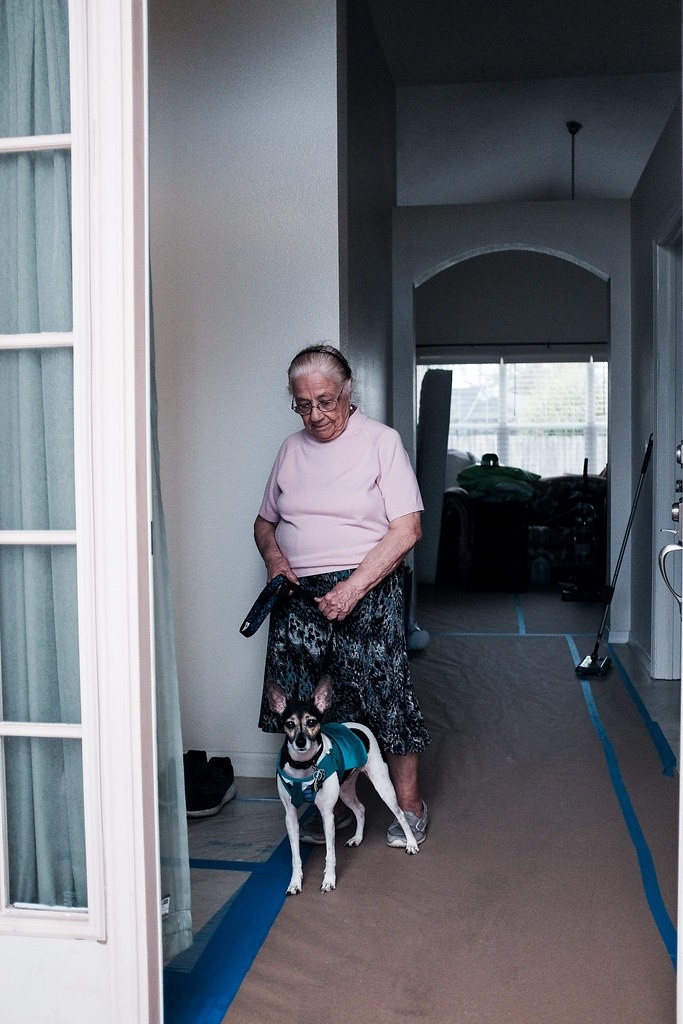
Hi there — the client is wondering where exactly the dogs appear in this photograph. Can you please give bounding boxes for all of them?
[267,673,421,897]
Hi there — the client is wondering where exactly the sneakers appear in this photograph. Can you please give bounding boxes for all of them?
[299,810,354,844]
[386,799,428,848]
[159,749,208,807]
[185,756,238,817]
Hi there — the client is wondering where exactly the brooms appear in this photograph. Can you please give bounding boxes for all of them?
[573,431,654,682]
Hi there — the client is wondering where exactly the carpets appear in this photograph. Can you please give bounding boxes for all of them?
[161,792,312,1024]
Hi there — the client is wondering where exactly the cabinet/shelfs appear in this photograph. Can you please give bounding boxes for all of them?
[417,369,451,582]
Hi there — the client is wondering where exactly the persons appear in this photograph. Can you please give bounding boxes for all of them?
[252,344,431,851]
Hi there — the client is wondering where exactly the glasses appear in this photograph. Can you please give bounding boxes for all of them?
[291,383,347,417]
